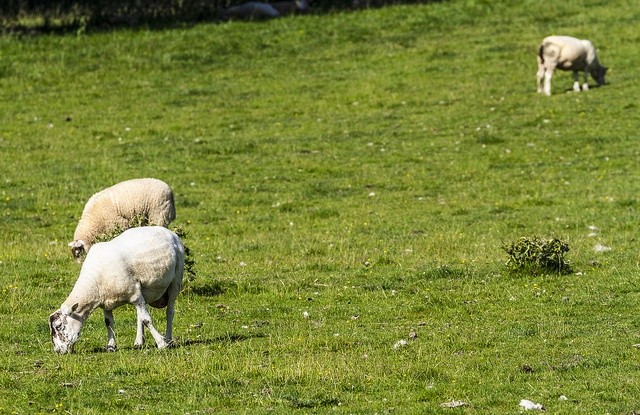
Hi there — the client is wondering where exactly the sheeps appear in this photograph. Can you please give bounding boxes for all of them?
[536,35,609,97]
[67,177,176,265]
[48,226,185,354]
